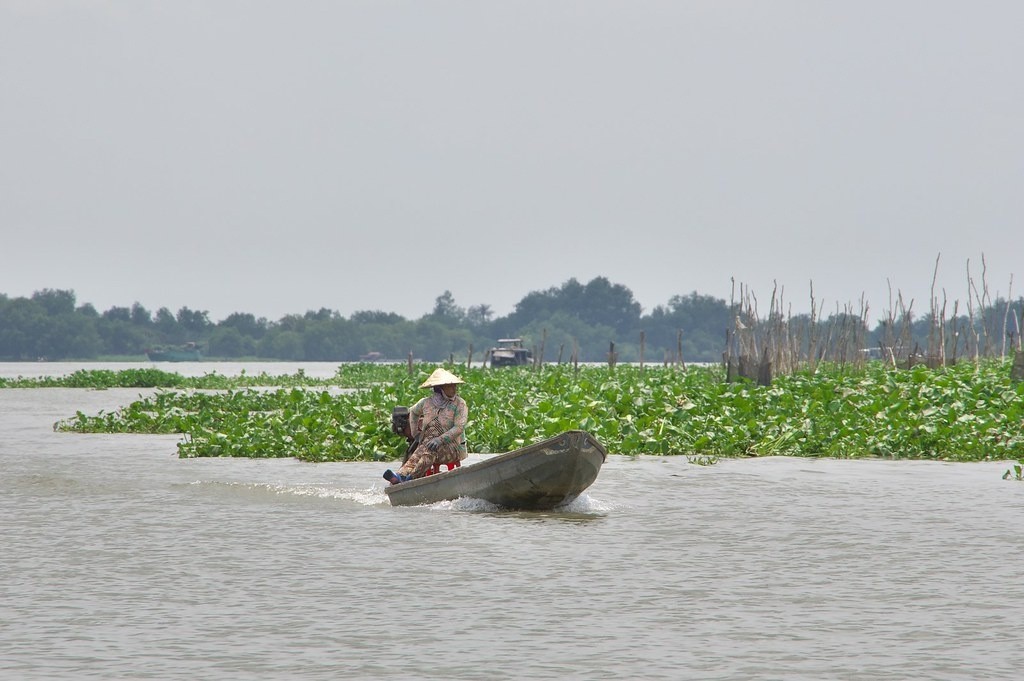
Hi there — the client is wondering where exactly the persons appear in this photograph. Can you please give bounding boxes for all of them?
[383,368,469,485]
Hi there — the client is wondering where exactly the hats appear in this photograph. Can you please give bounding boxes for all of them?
[419,368,466,389]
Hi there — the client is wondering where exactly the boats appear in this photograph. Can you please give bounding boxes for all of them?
[384,406,607,511]
[490,338,534,368]
[146,342,205,364]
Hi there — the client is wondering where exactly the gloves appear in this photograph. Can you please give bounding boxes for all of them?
[415,434,419,442]
[425,438,443,452]
